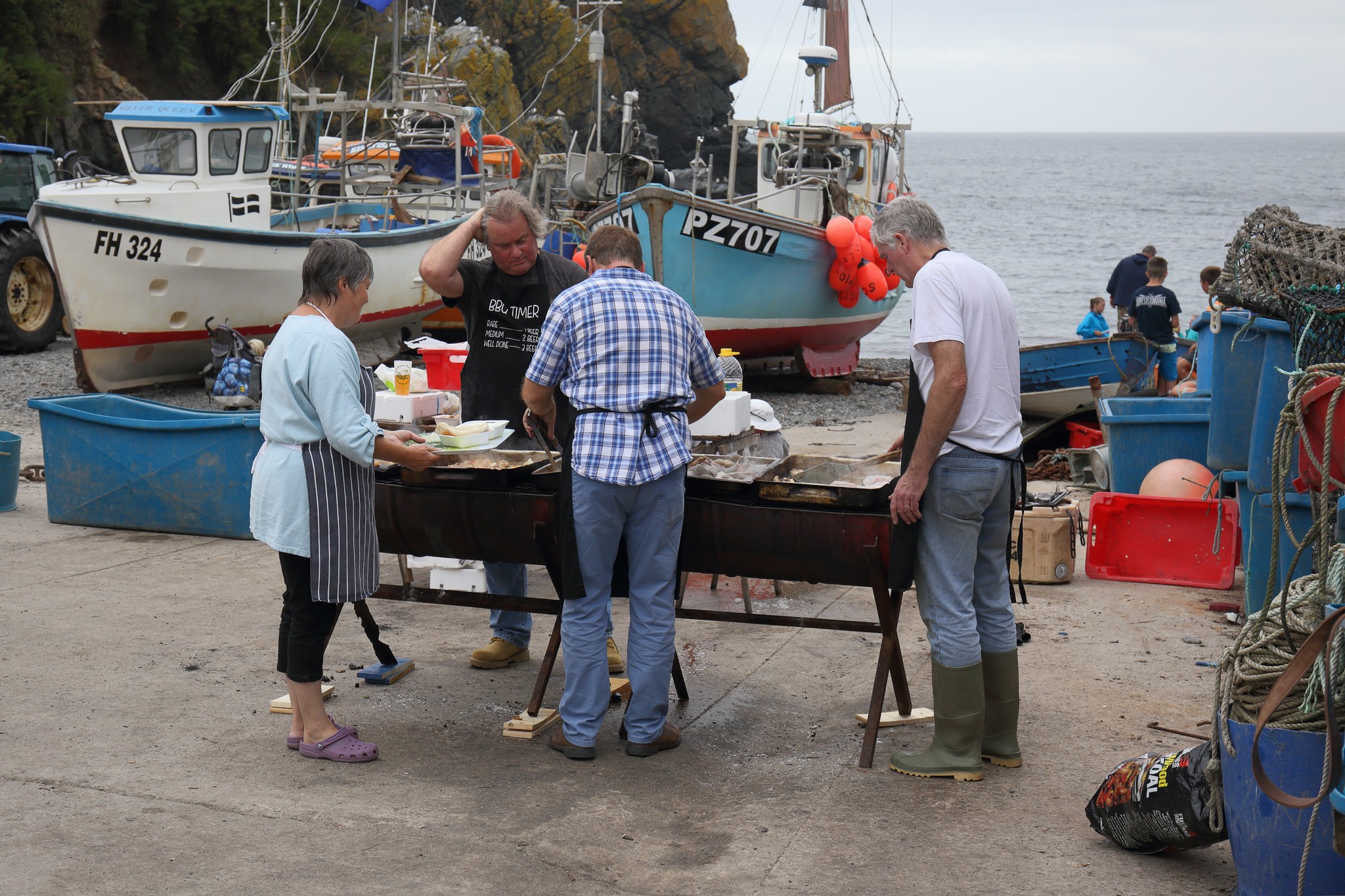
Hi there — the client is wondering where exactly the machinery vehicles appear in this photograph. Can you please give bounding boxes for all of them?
[0,143,66,355]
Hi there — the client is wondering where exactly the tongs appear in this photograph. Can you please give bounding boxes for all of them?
[526,409,563,470]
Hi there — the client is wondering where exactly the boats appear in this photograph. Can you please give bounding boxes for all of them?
[1015,331,1198,420]
[23,0,917,394]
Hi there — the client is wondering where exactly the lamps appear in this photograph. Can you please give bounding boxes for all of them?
[860,123,873,134]
[758,120,767,131]
[805,65,815,77]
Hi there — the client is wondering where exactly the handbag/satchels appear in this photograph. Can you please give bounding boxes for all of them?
[212,358,260,408]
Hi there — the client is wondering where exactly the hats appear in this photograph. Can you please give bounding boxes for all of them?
[750,398,782,432]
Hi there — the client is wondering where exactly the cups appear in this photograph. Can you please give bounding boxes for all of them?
[394,360,412,395]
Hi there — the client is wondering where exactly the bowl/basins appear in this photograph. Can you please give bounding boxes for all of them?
[434,418,459,426]
[435,420,509,448]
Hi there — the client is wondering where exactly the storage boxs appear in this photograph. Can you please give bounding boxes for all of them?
[417,348,469,390]
[28,393,268,540]
[1008,497,1085,581]
[373,389,445,424]
[1066,311,1345,620]
[431,414,460,426]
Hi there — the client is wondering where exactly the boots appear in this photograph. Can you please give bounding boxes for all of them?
[889,657,987,783]
[979,647,1025,767]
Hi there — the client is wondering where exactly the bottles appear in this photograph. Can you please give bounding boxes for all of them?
[717,348,743,391]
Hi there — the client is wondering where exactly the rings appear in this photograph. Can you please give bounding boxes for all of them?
[903,504,910,506]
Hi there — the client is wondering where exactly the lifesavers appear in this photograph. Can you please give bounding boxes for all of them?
[469,135,520,179]
[887,181,897,203]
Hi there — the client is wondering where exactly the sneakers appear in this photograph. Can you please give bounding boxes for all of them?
[625,720,682,757]
[606,637,624,673]
[468,635,530,669]
[550,725,597,759]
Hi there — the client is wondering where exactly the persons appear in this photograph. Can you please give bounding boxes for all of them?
[1177,342,1198,382]
[247,237,444,763]
[1128,256,1182,394]
[1077,297,1110,340]
[520,227,727,759]
[870,196,1024,781]
[1173,315,1198,341]
[1189,266,1243,327]
[420,187,625,673]
[1106,246,1157,332]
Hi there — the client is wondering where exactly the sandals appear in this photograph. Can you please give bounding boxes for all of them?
[299,727,379,762]
[286,714,359,750]
